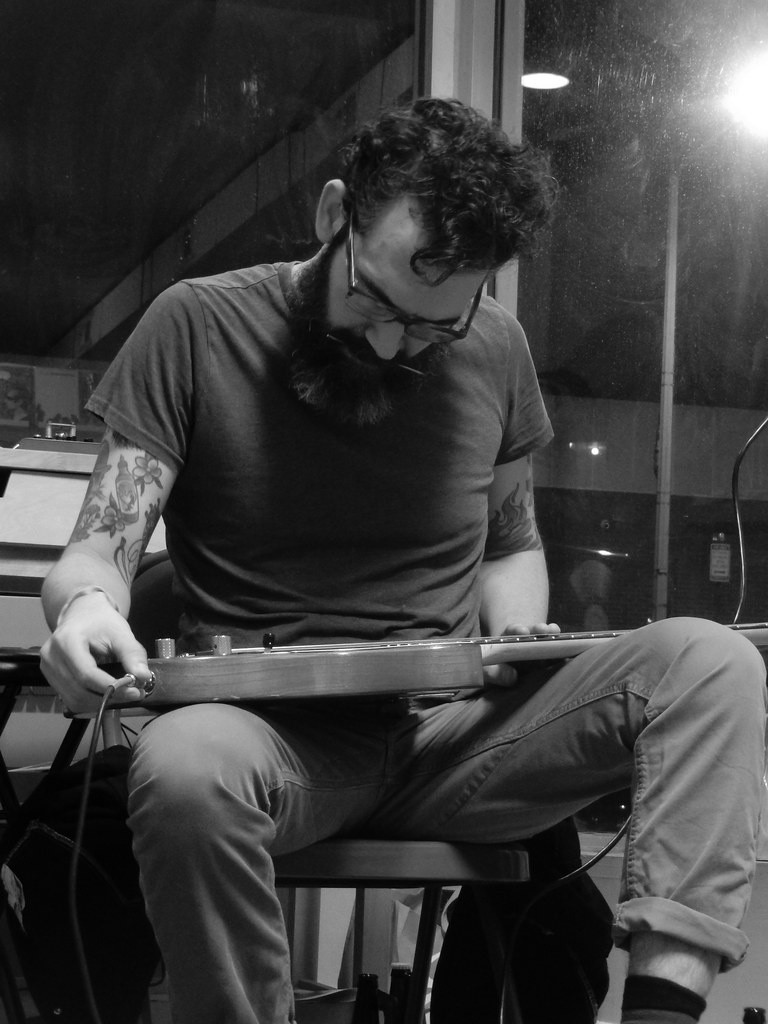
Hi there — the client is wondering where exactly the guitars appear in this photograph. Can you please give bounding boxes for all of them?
[126,616,768,701]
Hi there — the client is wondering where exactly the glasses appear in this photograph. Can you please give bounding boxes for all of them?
[345,214,482,344]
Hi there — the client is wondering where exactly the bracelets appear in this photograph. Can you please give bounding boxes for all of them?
[57,587,120,624]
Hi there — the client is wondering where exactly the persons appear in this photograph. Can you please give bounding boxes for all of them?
[41,96,768,1024]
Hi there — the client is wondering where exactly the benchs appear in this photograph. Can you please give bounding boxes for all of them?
[0,447,531,1024]
